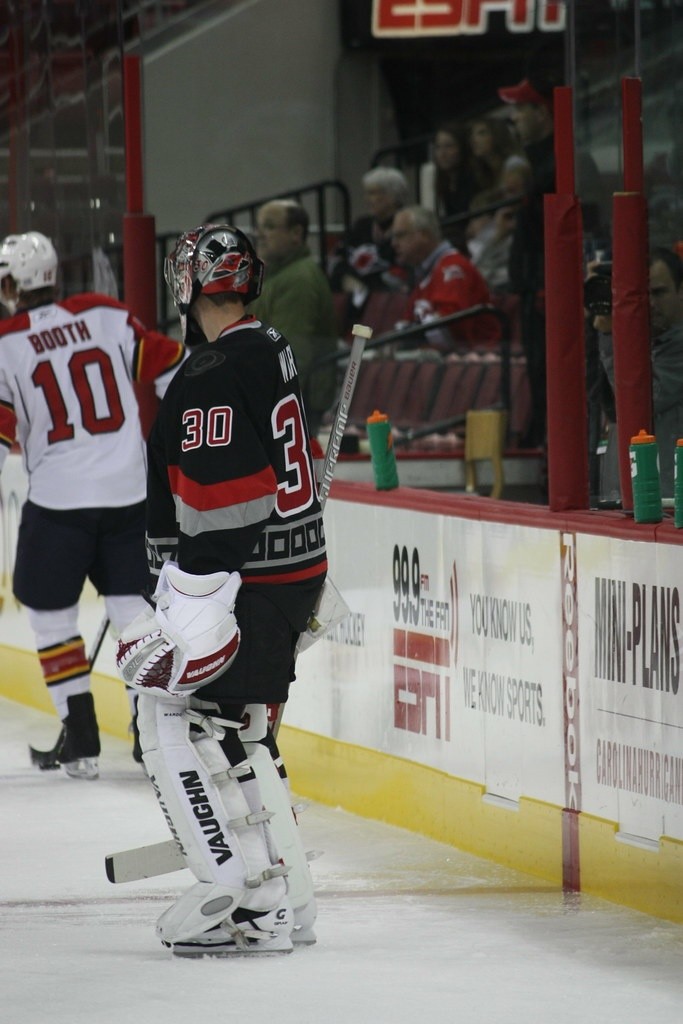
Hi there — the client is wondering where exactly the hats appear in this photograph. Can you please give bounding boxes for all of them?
[499,80,549,110]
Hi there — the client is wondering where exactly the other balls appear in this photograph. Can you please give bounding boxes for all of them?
[39,763,61,769]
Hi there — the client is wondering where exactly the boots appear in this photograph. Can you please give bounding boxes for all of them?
[57,691,101,780]
[129,694,149,779]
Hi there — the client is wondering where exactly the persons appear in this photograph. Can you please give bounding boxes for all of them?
[0,232,190,778]
[119,226,327,959]
[242,73,683,501]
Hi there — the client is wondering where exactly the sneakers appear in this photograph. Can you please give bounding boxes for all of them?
[290,895,317,947]
[173,896,294,959]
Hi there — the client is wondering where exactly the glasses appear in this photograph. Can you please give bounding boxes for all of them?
[386,227,413,240]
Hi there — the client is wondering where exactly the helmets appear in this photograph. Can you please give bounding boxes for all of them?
[0,231,58,314]
[169,224,264,313]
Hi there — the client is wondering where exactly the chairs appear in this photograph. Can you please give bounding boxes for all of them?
[333,295,536,449]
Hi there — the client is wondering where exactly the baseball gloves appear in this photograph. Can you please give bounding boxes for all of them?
[116,567,242,697]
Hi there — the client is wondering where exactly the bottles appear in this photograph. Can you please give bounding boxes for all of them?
[629,429,663,524]
[674,438,683,528]
[367,408,399,491]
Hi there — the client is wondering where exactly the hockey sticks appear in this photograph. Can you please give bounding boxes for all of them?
[104,323,374,884]
[26,610,110,763]
